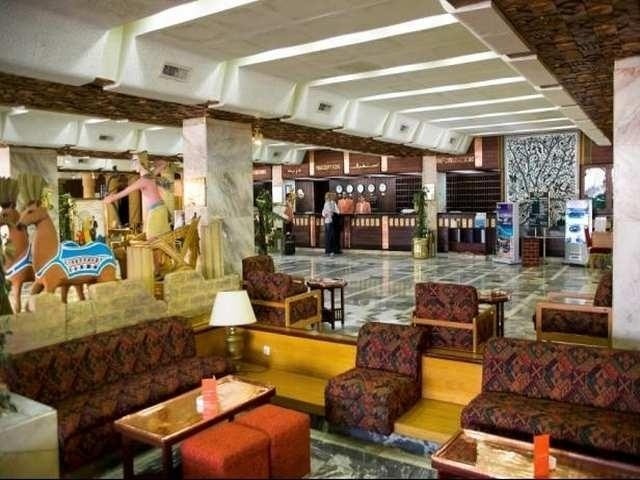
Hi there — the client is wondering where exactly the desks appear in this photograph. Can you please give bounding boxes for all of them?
[431,427,640,479]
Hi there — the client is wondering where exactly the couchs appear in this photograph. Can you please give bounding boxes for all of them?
[459,336,640,455]
[0,315,227,479]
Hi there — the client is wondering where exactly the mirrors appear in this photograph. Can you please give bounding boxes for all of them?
[580,164,614,215]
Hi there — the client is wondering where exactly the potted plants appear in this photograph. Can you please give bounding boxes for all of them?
[410,187,429,259]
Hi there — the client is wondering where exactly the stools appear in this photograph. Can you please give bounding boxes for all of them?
[233,404,312,480]
[180,418,271,480]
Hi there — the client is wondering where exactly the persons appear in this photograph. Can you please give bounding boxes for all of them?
[337,191,354,215]
[321,191,344,256]
[281,192,297,244]
[354,193,372,215]
[104,151,171,282]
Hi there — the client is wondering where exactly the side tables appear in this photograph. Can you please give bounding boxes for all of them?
[476,288,513,337]
[307,276,348,330]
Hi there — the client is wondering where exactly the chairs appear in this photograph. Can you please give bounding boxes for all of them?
[247,254,322,332]
[585,226,612,272]
[323,321,428,436]
[242,255,307,294]
[410,282,497,352]
[532,268,612,348]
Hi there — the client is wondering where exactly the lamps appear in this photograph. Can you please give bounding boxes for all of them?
[208,290,258,376]
